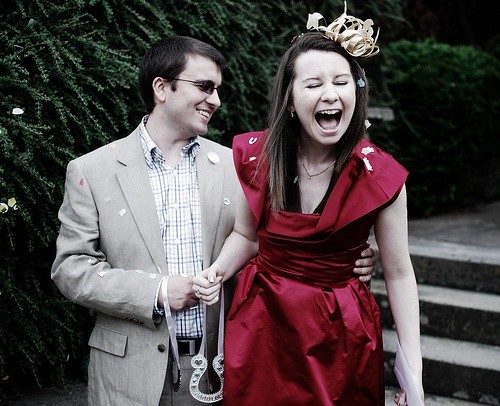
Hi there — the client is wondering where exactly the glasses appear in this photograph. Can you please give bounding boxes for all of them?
[174,78,228,99]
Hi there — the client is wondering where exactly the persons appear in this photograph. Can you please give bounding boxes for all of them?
[51,37,377,406]
[192,2,425,406]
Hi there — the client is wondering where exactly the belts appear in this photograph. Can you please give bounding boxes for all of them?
[169,338,202,356]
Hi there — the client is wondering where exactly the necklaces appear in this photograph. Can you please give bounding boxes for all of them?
[296,146,335,178]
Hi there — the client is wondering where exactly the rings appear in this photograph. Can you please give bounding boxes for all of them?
[200,295,204,301]
[197,286,201,295]
